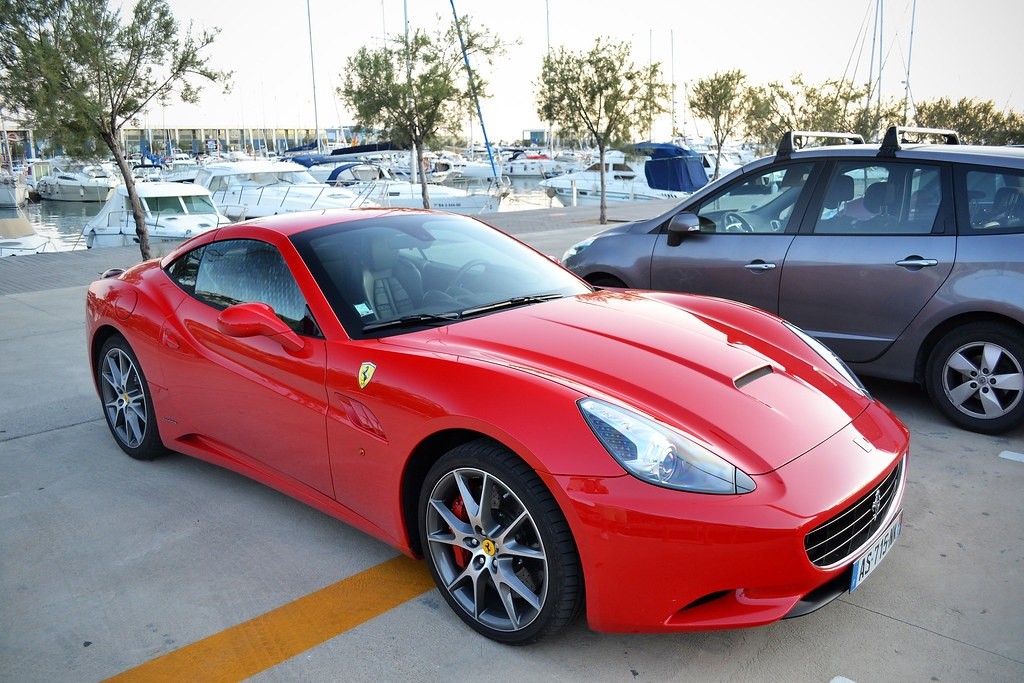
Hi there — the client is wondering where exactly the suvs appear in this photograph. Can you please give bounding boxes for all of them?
[558,120,1024,439]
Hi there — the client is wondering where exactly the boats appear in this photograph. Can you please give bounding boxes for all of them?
[0,133,905,250]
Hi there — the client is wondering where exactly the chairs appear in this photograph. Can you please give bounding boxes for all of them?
[814,173,858,233]
[862,182,900,234]
[360,235,426,320]
[994,185,1021,227]
[967,188,997,229]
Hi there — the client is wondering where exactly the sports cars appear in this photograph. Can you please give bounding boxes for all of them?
[82,203,917,651]
